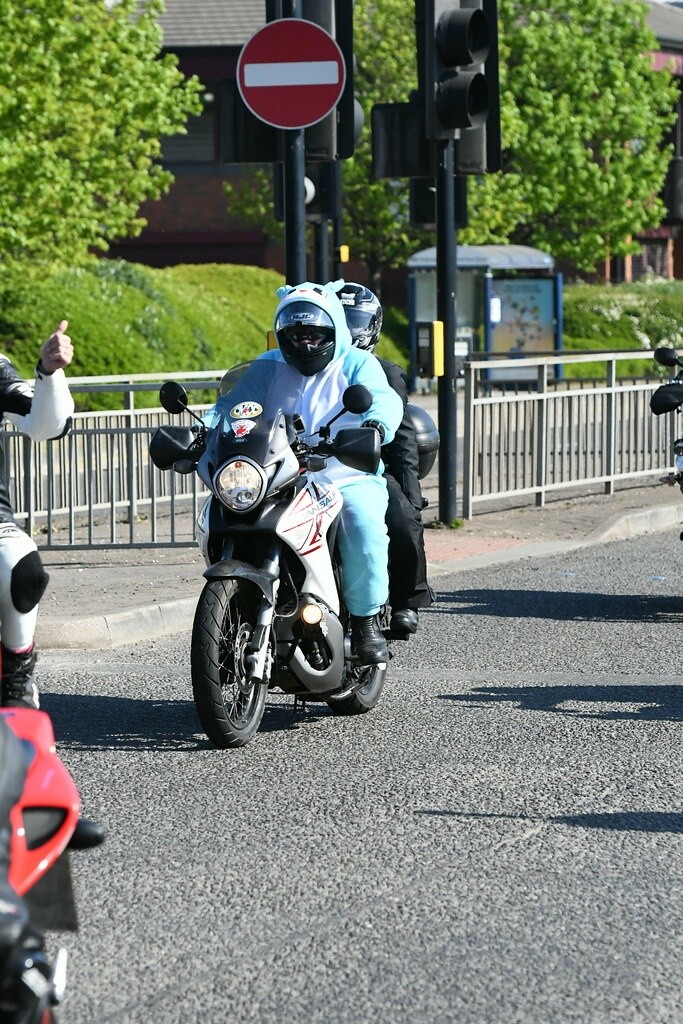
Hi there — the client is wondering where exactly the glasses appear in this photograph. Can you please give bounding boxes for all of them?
[284,326,327,341]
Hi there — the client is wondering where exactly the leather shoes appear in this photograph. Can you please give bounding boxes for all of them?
[350,614,390,664]
[389,606,418,634]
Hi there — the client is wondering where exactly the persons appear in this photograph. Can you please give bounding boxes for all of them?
[338,282,437,635]
[0,320,76,711]
[189,280,405,665]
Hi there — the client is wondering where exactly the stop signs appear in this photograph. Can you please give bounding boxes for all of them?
[233,16,346,131]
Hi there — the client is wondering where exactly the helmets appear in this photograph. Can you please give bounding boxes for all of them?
[274,281,346,378]
[332,282,383,354]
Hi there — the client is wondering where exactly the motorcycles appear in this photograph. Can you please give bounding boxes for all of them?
[650,347,683,542]
[141,374,439,751]
[0,697,107,1024]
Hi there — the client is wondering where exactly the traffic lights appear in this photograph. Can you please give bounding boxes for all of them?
[422,0,498,141]
